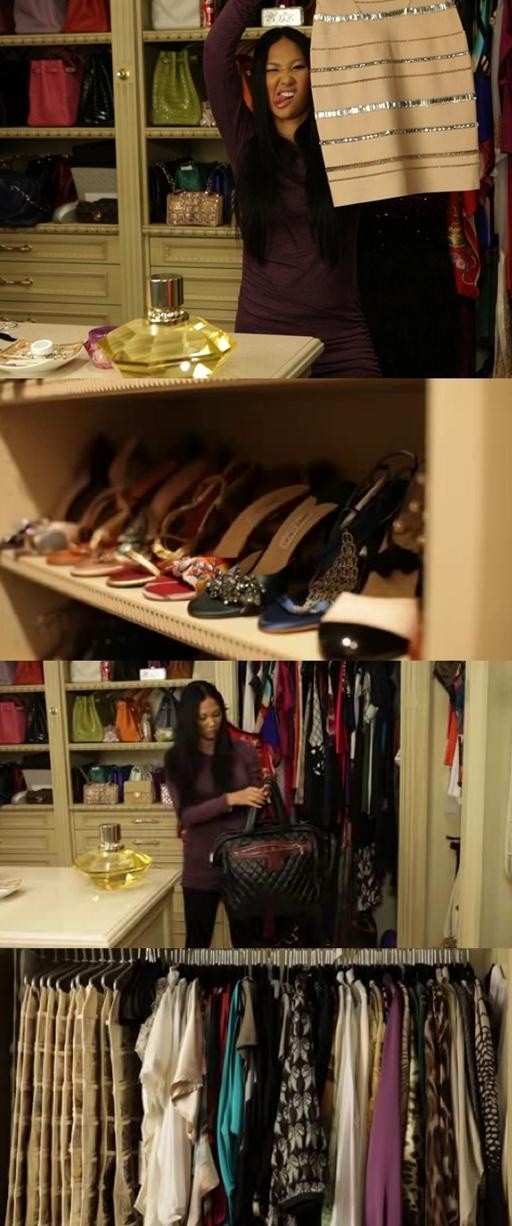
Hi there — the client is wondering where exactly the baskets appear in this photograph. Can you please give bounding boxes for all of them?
[261,4,304,27]
[165,189,225,229]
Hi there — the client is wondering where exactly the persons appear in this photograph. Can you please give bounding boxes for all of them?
[202,0,384,379]
[164,680,275,948]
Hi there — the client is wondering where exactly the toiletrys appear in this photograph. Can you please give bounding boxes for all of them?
[74,821,155,893]
[95,274,238,379]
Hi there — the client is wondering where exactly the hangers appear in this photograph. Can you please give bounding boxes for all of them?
[16,949,167,992]
[166,948,470,968]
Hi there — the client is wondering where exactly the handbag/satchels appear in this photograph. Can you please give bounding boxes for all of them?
[73,761,96,803]
[27,48,84,127]
[209,778,338,923]
[76,52,114,128]
[69,693,116,743]
[114,699,141,742]
[88,765,113,783]
[108,766,124,804]
[150,1,202,31]
[69,167,117,199]
[3,155,52,225]
[142,711,152,742]
[129,765,144,782]
[0,695,29,744]
[123,780,156,804]
[204,163,232,227]
[74,198,118,224]
[12,0,63,36]
[25,701,48,743]
[65,2,112,34]
[146,160,173,224]
[151,50,202,127]
[32,151,78,204]
[204,2,219,31]
[153,768,166,802]
[143,771,153,782]
[149,687,179,729]
[82,782,119,806]
[200,97,218,127]
[65,141,115,168]
[174,158,216,189]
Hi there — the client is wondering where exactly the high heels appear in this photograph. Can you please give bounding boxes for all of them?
[48,433,181,566]
[24,435,142,557]
[142,479,314,601]
[107,470,231,588]
[317,461,426,661]
[1,431,118,549]
[70,457,216,578]
[188,457,358,618]
[259,447,419,634]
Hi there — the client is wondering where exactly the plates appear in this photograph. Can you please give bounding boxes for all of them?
[0,339,86,374]
[0,876,24,899]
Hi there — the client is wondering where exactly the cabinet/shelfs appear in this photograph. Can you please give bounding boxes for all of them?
[173,890,228,949]
[0,809,54,865]
[129,0,310,231]
[72,809,185,866]
[0,234,126,324]
[142,234,244,332]
[1,381,512,660]
[0,661,55,808]
[59,661,234,806]
[0,1,125,230]
